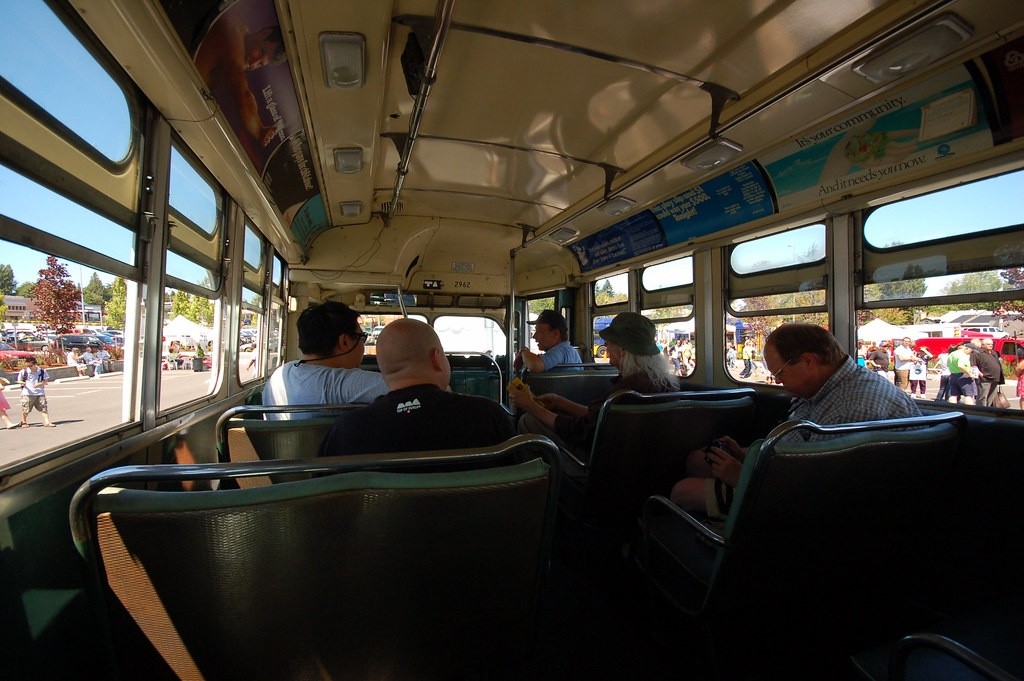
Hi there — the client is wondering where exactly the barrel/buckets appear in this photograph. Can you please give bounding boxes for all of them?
[193,358,203,372]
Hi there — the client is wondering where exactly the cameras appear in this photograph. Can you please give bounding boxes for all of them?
[704,441,737,468]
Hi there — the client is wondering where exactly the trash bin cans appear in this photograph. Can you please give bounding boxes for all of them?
[192,357,203,371]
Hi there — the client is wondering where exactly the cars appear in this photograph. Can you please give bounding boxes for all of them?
[239,327,279,352]
[0,327,125,363]
[361,332,375,345]
[373,326,385,337]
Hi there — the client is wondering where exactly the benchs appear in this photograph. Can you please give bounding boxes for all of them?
[70,351,1024,681]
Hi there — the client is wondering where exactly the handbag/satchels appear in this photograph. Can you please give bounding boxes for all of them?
[992,385,1010,409]
[908,360,926,381]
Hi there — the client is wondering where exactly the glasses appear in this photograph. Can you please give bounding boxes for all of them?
[354,332,368,343]
[770,358,792,380]
[26,363,34,367]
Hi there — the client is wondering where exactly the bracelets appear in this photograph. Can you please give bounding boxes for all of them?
[519,349,525,355]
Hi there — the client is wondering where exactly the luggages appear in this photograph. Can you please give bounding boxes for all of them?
[680,360,687,376]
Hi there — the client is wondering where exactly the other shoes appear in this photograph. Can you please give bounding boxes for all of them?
[7,422,19,429]
[920,394,926,399]
[18,421,29,427]
[43,422,56,427]
[911,394,916,399]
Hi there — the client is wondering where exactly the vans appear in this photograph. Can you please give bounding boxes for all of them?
[961,326,1009,339]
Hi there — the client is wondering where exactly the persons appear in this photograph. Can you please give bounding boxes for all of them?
[935,339,1005,407]
[514,309,584,374]
[312,319,524,478]
[1015,349,1024,410]
[0,377,20,430]
[654,335,696,377]
[75,347,103,376]
[169,349,183,369]
[726,337,758,378]
[893,337,934,399]
[669,321,924,521]
[247,342,257,379]
[97,346,114,373]
[67,347,87,377]
[17,357,56,428]
[509,311,682,463]
[858,339,889,381]
[261,299,453,421]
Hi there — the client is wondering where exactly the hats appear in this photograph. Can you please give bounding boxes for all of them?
[526,309,568,329]
[879,342,886,346]
[599,311,660,356]
[965,342,977,349]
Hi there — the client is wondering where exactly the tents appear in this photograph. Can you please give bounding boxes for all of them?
[658,317,737,351]
[858,318,928,361]
[162,315,213,352]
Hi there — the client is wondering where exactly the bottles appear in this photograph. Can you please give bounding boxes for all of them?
[507,377,546,411]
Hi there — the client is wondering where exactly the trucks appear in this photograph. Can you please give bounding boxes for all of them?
[406,311,547,366]
[914,336,1024,366]
[593,317,614,358]
